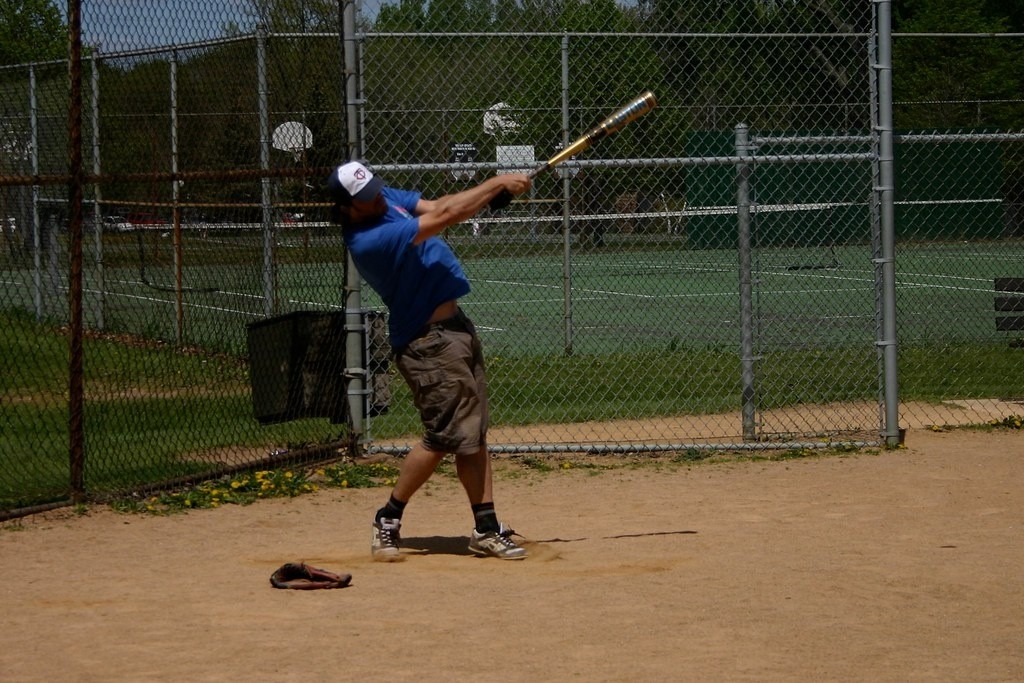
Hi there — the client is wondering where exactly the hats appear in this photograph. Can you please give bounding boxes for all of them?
[329,160,386,201]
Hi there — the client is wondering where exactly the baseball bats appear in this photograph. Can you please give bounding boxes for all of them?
[486,88,658,212]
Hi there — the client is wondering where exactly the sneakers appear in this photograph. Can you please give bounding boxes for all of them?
[468,522,528,559]
[371,505,401,561]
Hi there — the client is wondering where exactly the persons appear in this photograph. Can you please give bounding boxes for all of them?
[328,159,531,562]
[580,150,607,248]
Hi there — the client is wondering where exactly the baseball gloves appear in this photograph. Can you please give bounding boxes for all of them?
[269,561,353,591]
